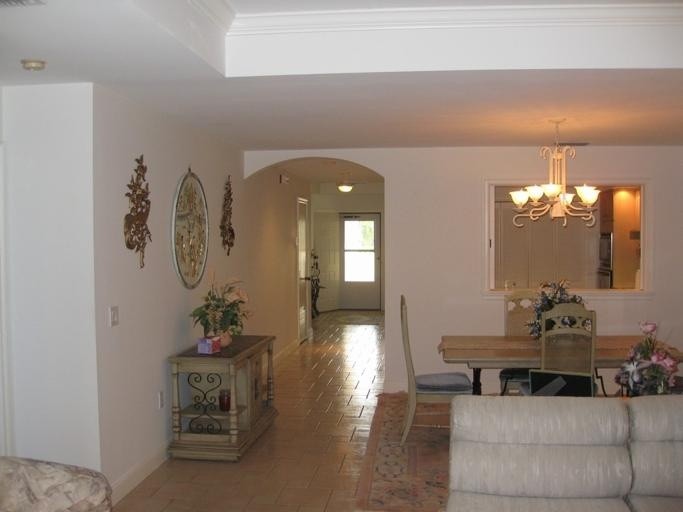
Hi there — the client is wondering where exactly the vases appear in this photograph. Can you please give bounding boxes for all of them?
[208,330,232,347]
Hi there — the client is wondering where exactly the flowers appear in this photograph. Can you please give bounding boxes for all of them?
[614,322,679,394]
[526,280,591,342]
[190,273,253,335]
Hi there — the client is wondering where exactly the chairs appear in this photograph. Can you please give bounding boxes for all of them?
[499,290,540,396]
[0,456,112,512]
[399,295,471,448]
[540,303,596,375]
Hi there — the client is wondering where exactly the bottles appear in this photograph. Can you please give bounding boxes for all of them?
[220,390,230,411]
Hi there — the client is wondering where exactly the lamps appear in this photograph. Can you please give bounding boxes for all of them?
[509,119,601,228]
[337,184,353,192]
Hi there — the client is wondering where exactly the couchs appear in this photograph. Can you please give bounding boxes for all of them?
[447,396,683,512]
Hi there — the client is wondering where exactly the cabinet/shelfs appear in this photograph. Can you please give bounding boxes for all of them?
[496,186,601,288]
[169,335,281,462]
